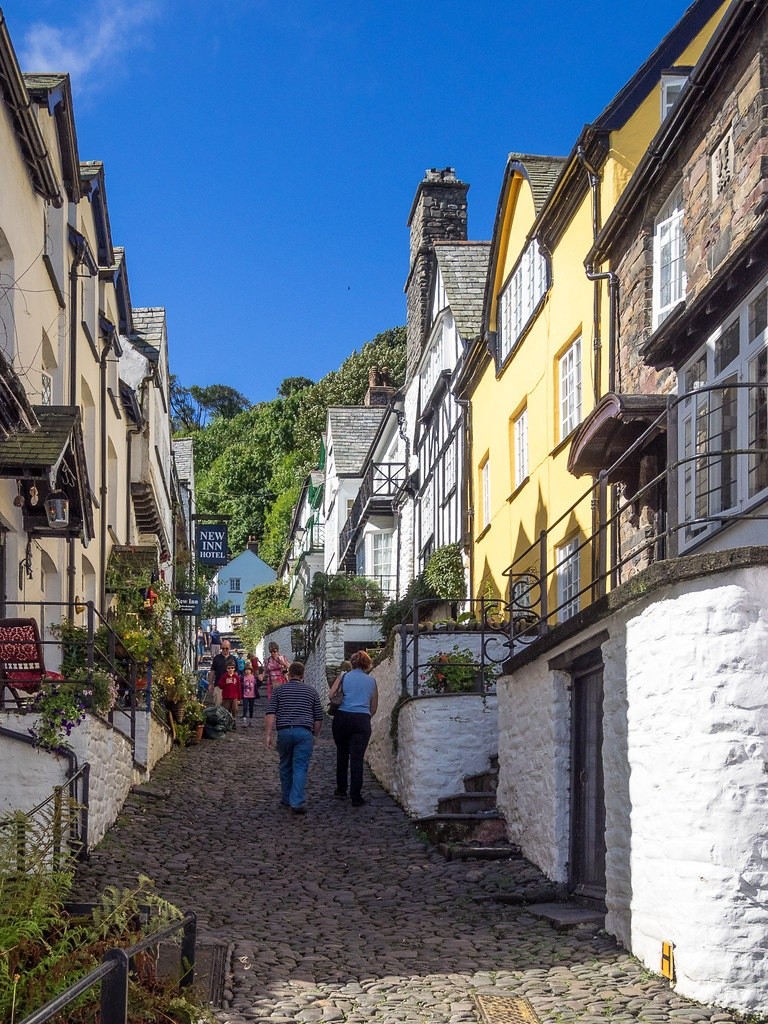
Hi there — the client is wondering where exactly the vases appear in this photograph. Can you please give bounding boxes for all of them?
[444,672,482,694]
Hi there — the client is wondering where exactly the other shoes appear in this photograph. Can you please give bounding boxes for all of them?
[232,723,237,730]
[294,807,306,814]
[352,794,365,807]
[243,720,248,727]
[249,719,254,727]
[334,787,346,798]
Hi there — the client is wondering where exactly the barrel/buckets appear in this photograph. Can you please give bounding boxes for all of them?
[43,491,69,528]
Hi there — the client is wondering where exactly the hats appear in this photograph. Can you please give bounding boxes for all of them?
[245,662,252,669]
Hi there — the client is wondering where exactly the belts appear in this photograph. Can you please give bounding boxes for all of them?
[277,725,311,731]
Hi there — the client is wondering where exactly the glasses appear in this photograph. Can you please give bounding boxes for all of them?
[222,647,228,650]
[227,667,234,669]
[270,651,277,653]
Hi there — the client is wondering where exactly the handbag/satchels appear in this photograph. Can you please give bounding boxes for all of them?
[283,670,289,682]
[327,673,346,715]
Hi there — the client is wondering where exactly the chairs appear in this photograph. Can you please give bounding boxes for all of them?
[0,617,65,708]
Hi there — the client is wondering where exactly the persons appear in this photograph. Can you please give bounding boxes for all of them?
[207,625,222,664]
[264,661,324,813]
[197,627,208,663]
[208,639,291,730]
[326,649,378,806]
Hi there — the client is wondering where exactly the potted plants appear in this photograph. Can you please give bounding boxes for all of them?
[168,673,205,738]
[304,572,390,619]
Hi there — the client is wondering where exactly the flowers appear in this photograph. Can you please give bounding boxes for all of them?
[418,644,502,696]
